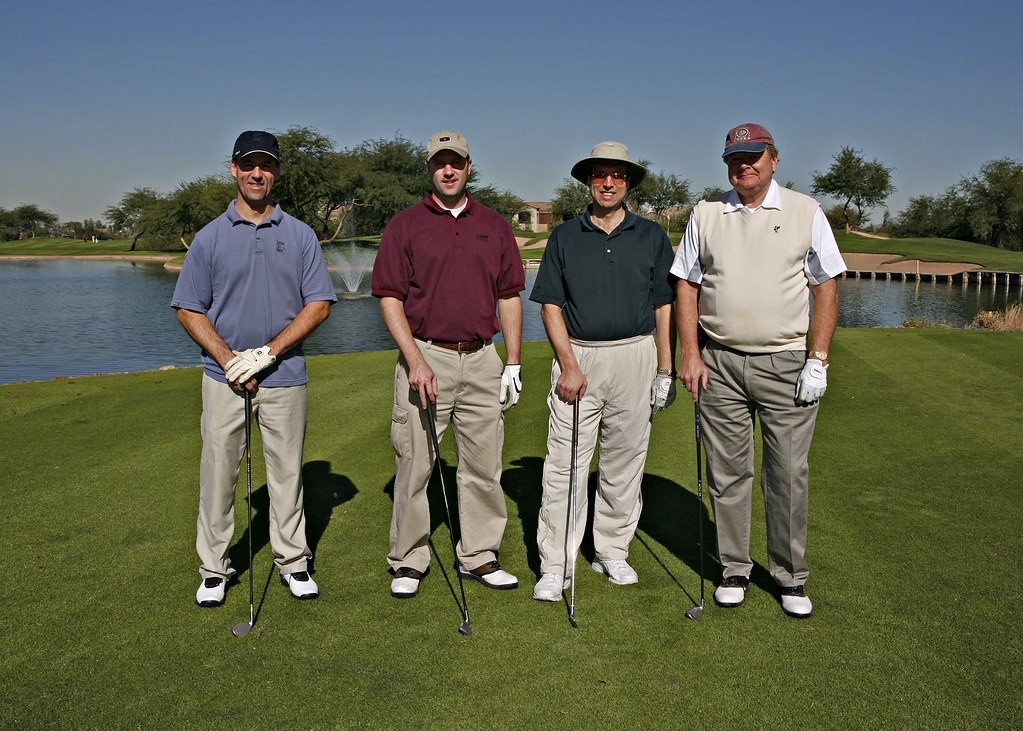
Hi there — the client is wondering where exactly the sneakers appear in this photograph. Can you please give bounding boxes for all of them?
[781,584,813,619]
[279,571,319,600]
[459,560,519,590]
[195,577,227,608]
[591,555,639,585]
[532,573,571,601]
[713,575,750,608]
[391,567,431,598]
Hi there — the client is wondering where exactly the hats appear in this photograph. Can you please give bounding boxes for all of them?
[569,141,646,191]
[721,123,774,158]
[232,130,280,163]
[424,130,470,162]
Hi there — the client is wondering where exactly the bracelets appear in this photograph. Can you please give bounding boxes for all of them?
[658,367,676,378]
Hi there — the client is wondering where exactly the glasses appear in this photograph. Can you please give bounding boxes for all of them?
[590,171,628,186]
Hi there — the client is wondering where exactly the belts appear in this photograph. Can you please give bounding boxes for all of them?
[420,338,494,355]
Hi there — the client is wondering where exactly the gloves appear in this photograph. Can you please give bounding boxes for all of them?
[499,363,523,412]
[795,359,829,407]
[224,345,277,384]
[651,374,673,415]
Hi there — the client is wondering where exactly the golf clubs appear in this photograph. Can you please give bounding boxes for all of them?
[424,395,470,634]
[567,393,580,629]
[232,389,255,638]
[685,377,706,621]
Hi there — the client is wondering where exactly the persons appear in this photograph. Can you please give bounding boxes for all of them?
[171,131,339,607]
[528,141,677,602]
[372,130,526,596]
[82,232,95,243]
[668,123,847,619]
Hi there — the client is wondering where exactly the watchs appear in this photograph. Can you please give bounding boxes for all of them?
[808,350,830,361]
